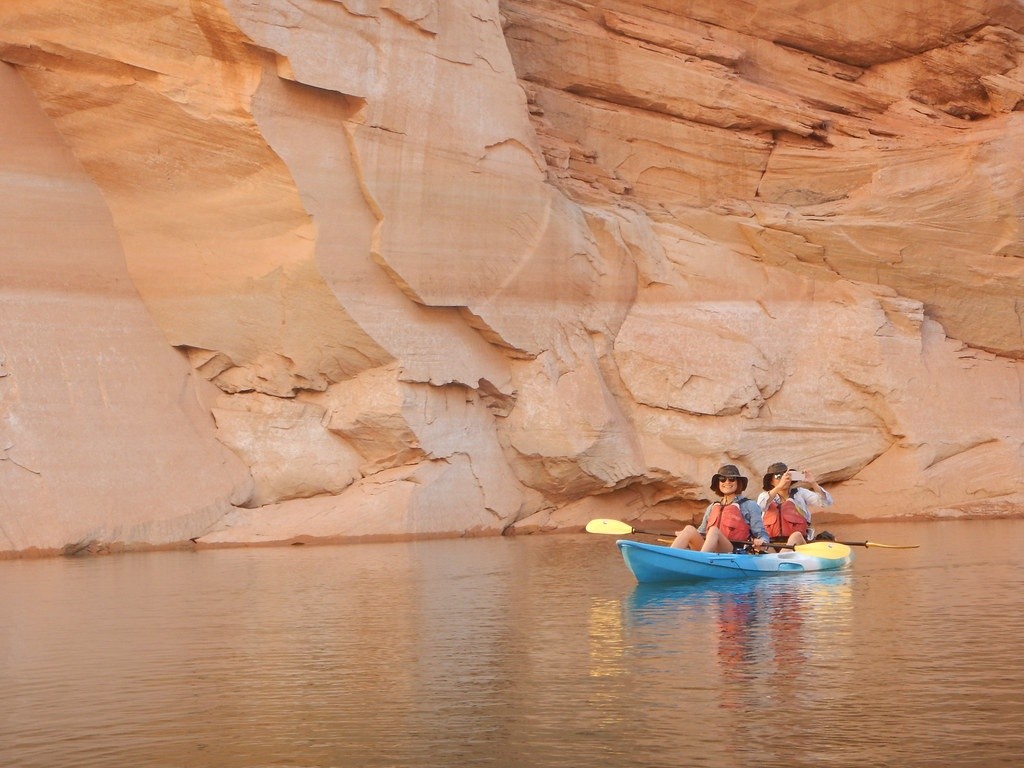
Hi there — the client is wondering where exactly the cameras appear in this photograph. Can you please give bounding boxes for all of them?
[788,470,805,481]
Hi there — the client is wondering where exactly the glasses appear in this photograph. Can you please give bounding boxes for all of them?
[775,473,783,479]
[718,476,737,482]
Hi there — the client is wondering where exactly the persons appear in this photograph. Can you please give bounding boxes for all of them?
[757,462,831,552]
[669,465,769,552]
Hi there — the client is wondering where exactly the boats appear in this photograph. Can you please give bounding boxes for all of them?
[615,539,857,583]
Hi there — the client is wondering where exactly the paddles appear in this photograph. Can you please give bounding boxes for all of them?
[584,517,853,561]
[769,539,921,549]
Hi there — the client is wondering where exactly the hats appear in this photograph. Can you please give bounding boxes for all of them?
[710,464,748,492]
[763,463,799,491]
